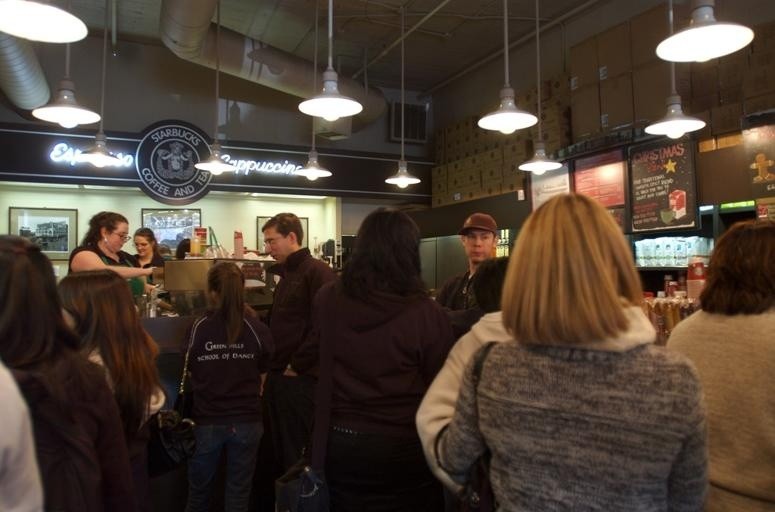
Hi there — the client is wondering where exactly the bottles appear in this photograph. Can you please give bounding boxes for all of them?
[644,274,689,343]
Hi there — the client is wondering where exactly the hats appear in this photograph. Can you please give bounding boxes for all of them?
[458,213,497,234]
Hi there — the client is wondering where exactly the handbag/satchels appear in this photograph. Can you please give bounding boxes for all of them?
[133,375,199,509]
[435,343,499,512]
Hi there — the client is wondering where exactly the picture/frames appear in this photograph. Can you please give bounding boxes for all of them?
[255,215,310,255]
[8,206,79,261]
[139,207,202,251]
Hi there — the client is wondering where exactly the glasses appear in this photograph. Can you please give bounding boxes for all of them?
[262,236,282,246]
[134,242,148,247]
[113,230,131,241]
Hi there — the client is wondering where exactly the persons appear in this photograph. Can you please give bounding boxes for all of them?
[174,264,269,510]
[1,234,129,511]
[261,212,337,509]
[175,238,190,262]
[666,220,774,510]
[437,212,497,335]
[439,192,711,510]
[131,228,163,271]
[57,269,165,511]
[70,210,156,314]
[418,255,637,510]
[1,364,44,511]
[309,208,454,512]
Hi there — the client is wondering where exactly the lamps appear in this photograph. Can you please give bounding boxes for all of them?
[80,0,119,169]
[0,0,89,45]
[518,1,564,177]
[384,5,422,189]
[477,1,538,136]
[292,1,332,181]
[194,0,236,176]
[298,0,363,123]
[643,0,707,141]
[32,0,102,129]
[656,2,756,64]
